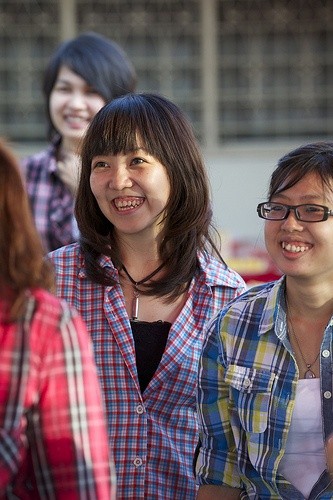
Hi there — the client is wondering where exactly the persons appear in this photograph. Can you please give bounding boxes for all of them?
[21,31,137,254]
[0,143,116,500]
[191,140,333,500]
[45,90,248,500]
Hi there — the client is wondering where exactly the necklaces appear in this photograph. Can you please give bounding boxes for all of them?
[115,256,167,320]
[286,299,320,380]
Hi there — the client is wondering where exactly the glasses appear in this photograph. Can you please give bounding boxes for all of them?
[257,202,333,222]
